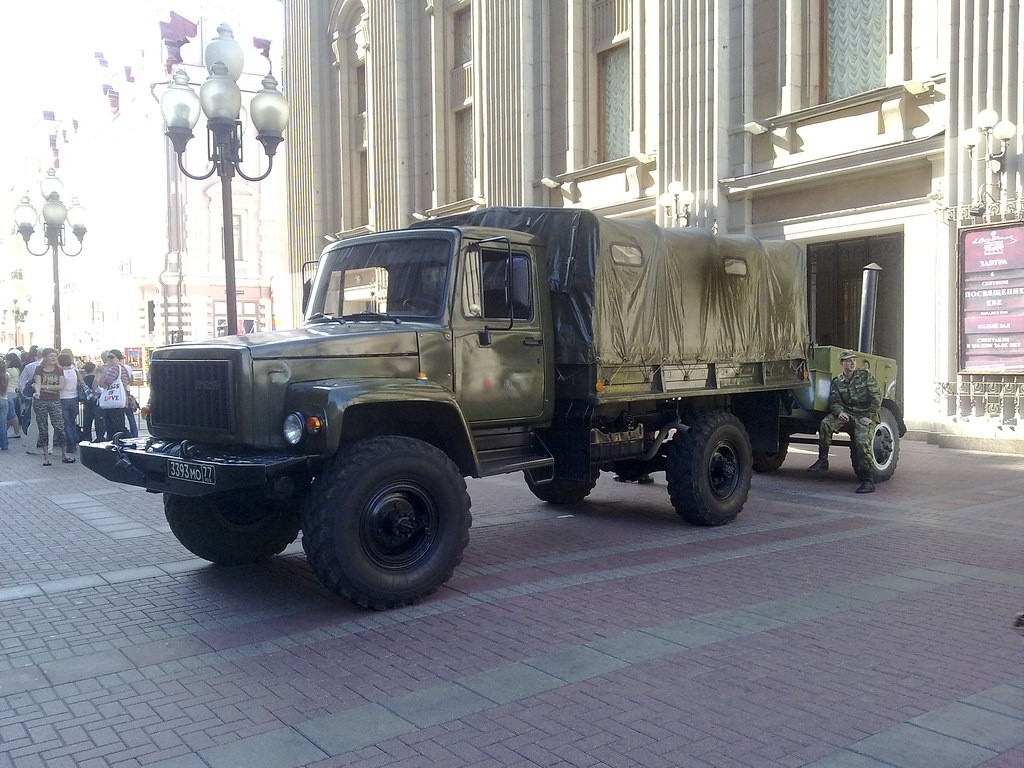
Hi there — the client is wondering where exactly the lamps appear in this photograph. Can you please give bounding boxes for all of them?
[966,180,1003,217]
[658,180,694,226]
[963,108,1017,171]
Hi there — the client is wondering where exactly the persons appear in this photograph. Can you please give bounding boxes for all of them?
[613,474,654,484]
[0,345,138,466]
[806,350,882,493]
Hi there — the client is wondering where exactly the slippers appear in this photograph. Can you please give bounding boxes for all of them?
[62,458,76,463]
[43,461,52,466]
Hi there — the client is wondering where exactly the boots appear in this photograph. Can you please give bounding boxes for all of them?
[856,472,875,493]
[808,446,829,472]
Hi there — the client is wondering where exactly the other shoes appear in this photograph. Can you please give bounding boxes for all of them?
[8,433,21,438]
[638,476,654,484]
[613,475,626,482]
[66,448,76,453]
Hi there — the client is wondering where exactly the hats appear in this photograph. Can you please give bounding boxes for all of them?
[109,349,122,359]
[101,350,109,363]
[36,347,43,353]
[838,351,858,361]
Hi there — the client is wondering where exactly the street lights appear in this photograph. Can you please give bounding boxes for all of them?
[14,167,88,353]
[152,20,288,341]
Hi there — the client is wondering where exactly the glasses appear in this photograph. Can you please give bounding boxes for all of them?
[107,355,115,359]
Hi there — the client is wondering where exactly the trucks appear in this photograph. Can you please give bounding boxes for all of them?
[78,204,813,614]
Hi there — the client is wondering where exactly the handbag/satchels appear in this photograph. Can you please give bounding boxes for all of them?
[128,394,140,412]
[100,365,126,409]
[23,379,36,397]
[77,383,92,402]
